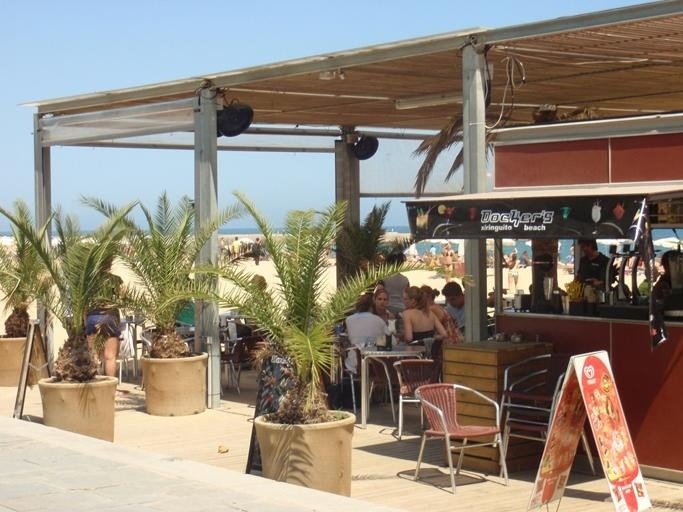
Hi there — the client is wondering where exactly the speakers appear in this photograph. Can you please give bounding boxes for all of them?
[217,103,253,136]
[353,136,378,160]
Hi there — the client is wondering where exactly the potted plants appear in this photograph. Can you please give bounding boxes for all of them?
[0,198,140,443]
[159,190,474,498]
[78,194,249,419]
[0,197,54,389]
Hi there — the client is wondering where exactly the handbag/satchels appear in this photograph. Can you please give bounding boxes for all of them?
[326,382,360,410]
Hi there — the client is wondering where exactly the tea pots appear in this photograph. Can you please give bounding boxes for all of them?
[509,328,523,343]
[494,328,507,343]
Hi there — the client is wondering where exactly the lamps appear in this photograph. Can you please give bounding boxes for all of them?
[351,133,380,159]
[211,98,253,137]
[388,95,467,110]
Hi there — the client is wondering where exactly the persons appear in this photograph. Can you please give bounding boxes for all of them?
[557,238,682,320]
[232,275,273,339]
[173,280,207,353]
[506,248,532,295]
[86,252,124,379]
[344,242,466,377]
[231,237,261,266]
